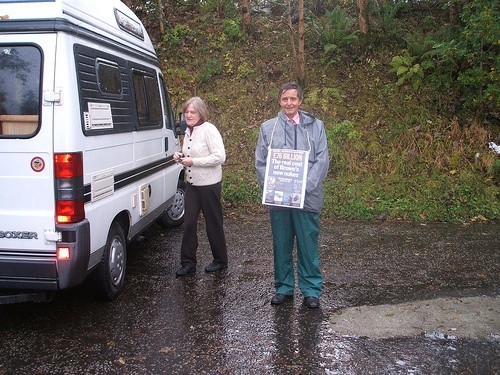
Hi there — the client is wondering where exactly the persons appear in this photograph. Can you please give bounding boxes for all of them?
[255,81,329,308]
[173,96,229,277]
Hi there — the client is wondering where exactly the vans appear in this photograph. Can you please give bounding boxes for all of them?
[0,0,187,298]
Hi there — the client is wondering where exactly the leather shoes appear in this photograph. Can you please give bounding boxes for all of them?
[205,261,229,272]
[271,294,293,304]
[304,296,320,308]
[176,266,196,275]
